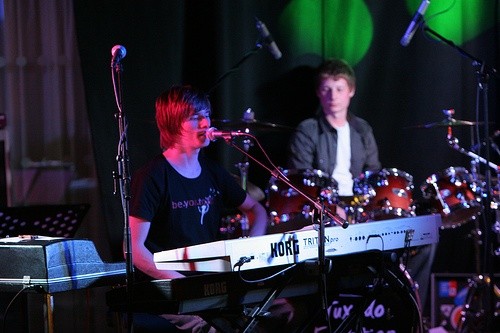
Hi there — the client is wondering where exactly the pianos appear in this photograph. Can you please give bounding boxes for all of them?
[152,214,444,333]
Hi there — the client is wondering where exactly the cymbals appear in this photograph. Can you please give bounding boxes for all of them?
[418,117,485,128]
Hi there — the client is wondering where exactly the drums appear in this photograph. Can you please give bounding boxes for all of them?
[352,168,418,221]
[264,167,339,231]
[419,165,485,230]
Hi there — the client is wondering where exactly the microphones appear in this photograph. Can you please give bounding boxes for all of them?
[109,45,127,68]
[205,126,245,142]
[255,19,283,60]
[400,0,430,47]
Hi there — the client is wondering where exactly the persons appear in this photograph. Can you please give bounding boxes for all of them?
[123,86,268,279]
[288,60,434,199]
